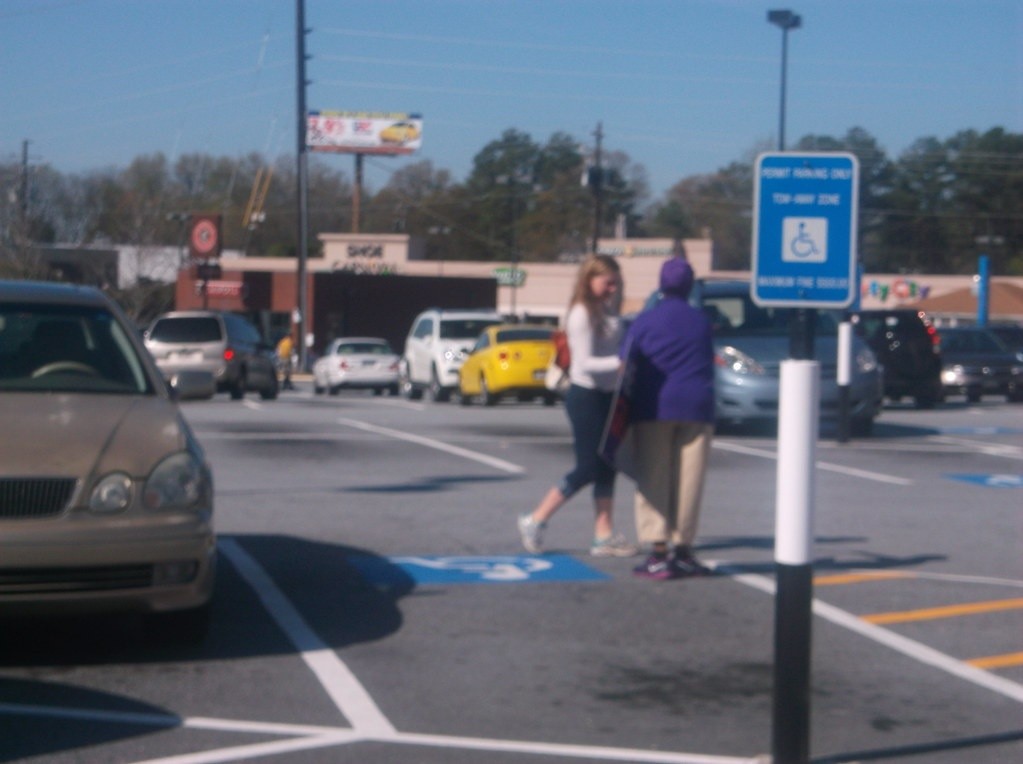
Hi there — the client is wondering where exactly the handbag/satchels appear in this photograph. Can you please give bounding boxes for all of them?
[555,331,570,372]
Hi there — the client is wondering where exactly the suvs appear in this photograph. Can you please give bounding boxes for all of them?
[845,308,940,408]
[401,309,506,401]
[645,274,888,438]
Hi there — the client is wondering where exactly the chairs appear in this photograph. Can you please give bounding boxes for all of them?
[703,305,732,326]
[27,319,110,377]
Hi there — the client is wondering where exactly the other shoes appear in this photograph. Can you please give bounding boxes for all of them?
[668,549,712,577]
[590,531,638,556]
[518,515,546,555]
[633,548,674,579]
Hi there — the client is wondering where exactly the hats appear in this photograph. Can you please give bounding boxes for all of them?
[659,257,694,288]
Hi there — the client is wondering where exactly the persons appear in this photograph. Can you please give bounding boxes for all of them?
[278,326,297,389]
[517,254,637,556]
[618,258,731,580]
[924,315,940,345]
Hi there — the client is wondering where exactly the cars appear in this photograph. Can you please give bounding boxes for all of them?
[927,329,1023,401]
[990,328,1023,359]
[461,323,558,405]
[312,337,400,396]
[0,278,217,623]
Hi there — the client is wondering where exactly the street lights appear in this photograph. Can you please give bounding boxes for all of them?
[768,8,800,150]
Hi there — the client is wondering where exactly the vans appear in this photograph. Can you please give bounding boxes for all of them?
[144,310,278,400]
